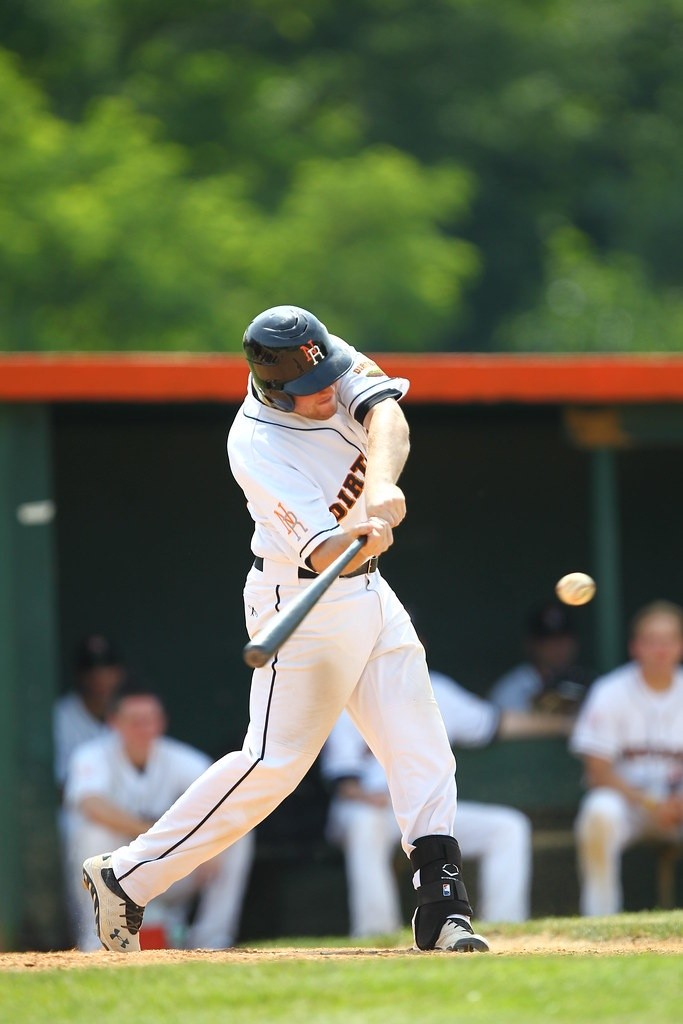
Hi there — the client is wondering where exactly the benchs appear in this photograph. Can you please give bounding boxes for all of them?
[450,729,683,907]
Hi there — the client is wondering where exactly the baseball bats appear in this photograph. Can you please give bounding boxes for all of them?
[238,532,371,669]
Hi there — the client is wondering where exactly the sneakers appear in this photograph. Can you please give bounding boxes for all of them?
[81,852,145,954]
[408,907,496,952]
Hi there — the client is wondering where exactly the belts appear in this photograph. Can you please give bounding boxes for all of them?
[255,556,378,580]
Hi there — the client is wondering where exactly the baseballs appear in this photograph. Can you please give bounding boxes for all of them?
[556,571,597,606]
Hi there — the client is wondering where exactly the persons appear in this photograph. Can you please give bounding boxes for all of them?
[319,666,576,937]
[568,601,683,917]
[51,653,126,797]
[81,305,493,951]
[55,684,252,949]
[485,605,577,740]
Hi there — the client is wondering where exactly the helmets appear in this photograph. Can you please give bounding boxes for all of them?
[244,306,353,411]
